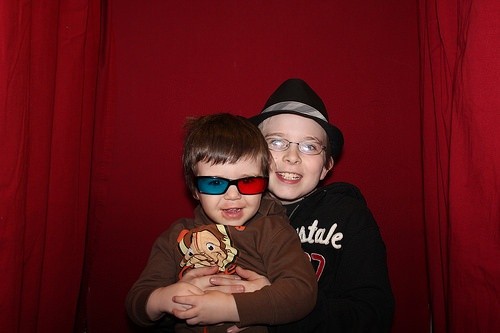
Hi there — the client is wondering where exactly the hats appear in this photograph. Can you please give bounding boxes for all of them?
[246,78,344,163]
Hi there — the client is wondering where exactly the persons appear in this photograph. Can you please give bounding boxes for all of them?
[125,112,319,333]
[179,77,396,333]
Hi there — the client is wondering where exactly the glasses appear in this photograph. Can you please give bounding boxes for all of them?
[195,176,270,194]
[266,137,329,156]
